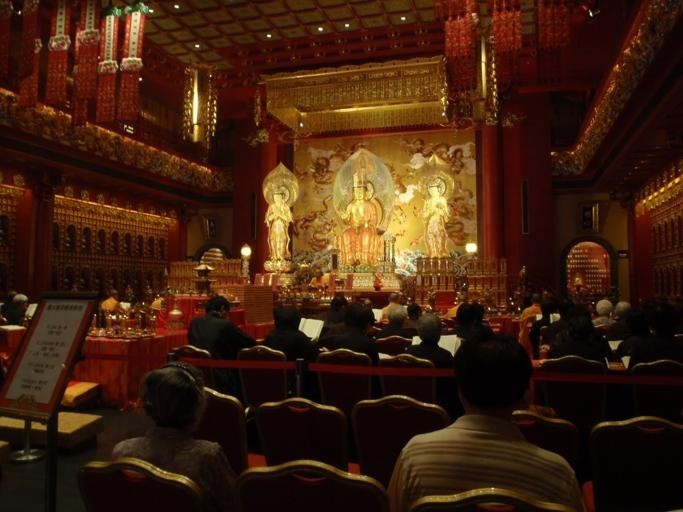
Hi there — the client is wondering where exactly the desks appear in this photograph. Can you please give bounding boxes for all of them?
[2,411,104,511]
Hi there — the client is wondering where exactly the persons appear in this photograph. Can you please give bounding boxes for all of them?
[187,295,257,402]
[342,181,379,267]
[113,361,240,512]
[270,292,682,425]
[423,183,450,260]
[100,289,125,315]
[7,293,28,325]
[0,291,17,318]
[386,332,582,512]
[120,284,140,306]
[152,294,164,310]
[264,188,292,262]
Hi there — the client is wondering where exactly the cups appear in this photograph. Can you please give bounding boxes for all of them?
[275,283,332,304]
[93,303,158,339]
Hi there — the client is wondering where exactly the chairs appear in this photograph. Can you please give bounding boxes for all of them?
[196,384,250,474]
[1,268,682,424]
[255,397,348,472]
[352,395,451,473]
[580,415,682,510]
[78,457,202,510]
[510,411,580,473]
[408,487,577,512]
[233,458,391,511]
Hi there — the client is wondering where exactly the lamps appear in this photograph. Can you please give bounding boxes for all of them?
[240,241,253,276]
